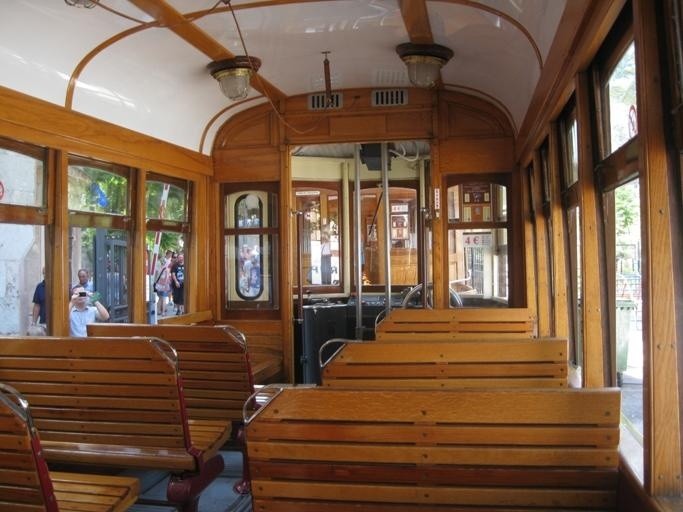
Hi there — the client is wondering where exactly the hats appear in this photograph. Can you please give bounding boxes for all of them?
[252,259,257,263]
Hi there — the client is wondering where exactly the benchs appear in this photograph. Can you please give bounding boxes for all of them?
[245,383,622,512]
[1,323,277,512]
[319,339,569,386]
[375,309,535,340]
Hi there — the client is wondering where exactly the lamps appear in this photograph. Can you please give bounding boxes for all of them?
[394,42,453,90]
[207,55,260,103]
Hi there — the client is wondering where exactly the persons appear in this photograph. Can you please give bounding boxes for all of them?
[153,249,184,316]
[30,266,46,327]
[361,236,366,272]
[320,231,333,285]
[69,269,110,338]
[239,243,261,297]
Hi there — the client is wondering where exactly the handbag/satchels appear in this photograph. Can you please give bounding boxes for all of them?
[153,282,157,292]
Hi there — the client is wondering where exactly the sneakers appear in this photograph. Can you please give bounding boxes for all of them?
[158,301,183,315]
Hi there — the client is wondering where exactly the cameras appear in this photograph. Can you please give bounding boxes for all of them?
[79,291,86,296]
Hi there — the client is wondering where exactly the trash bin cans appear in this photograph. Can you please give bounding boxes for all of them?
[159,291,170,316]
[150,293,158,324]
